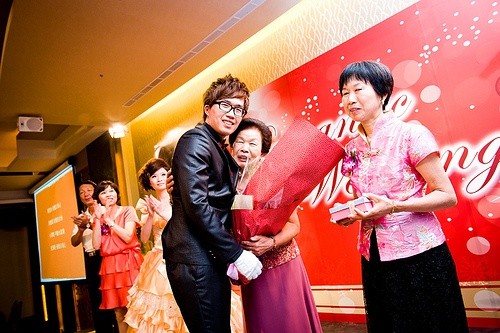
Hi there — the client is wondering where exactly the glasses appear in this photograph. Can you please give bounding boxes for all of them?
[212,100,248,117]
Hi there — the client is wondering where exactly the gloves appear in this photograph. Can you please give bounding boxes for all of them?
[233,249,263,280]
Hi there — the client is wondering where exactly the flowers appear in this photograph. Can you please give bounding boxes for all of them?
[342,150,361,175]
[101,223,111,236]
[155,218,168,228]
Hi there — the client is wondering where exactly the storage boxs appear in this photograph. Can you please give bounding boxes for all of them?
[329,196,373,221]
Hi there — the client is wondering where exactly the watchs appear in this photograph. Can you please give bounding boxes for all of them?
[86,222,90,229]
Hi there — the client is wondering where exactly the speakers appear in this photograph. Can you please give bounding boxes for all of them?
[17,116,44,132]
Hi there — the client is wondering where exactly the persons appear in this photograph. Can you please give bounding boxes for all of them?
[71,180,120,333]
[330,60,469,333]
[166,117,324,333]
[92,181,145,333]
[123,158,243,333]
[161,73,263,333]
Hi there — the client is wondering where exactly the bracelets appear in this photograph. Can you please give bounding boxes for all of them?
[109,223,115,228]
[389,200,395,215]
[270,236,276,251]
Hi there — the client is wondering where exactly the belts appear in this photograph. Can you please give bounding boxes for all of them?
[84,252,100,256]
